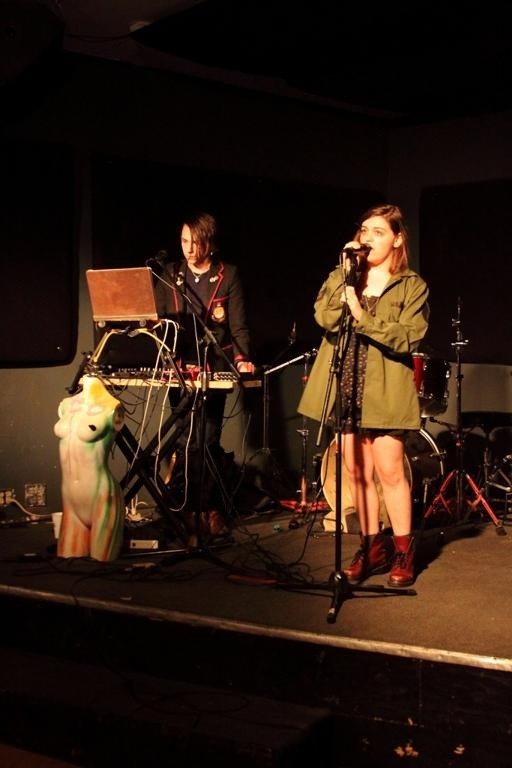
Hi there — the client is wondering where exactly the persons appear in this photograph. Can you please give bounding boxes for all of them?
[154,212,257,547]
[51,376,127,562]
[294,203,433,588]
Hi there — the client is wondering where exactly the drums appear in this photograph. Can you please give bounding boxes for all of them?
[412,353,450,419]
[320,427,445,529]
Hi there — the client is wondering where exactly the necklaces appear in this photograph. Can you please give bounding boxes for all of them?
[363,290,380,313]
[189,264,209,284]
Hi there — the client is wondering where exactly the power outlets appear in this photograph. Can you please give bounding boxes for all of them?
[0,488,16,508]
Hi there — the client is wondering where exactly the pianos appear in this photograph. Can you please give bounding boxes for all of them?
[78,362,263,393]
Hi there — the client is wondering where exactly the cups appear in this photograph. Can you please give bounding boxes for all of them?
[51,512,64,540]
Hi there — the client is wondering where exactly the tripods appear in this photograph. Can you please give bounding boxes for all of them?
[417,296,507,539]
[276,254,417,624]
[120,260,269,578]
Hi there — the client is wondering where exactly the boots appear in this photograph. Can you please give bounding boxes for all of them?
[388,534,417,587]
[342,530,389,579]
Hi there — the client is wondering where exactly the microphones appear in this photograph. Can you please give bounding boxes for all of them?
[344,242,372,254]
[146,250,168,261]
